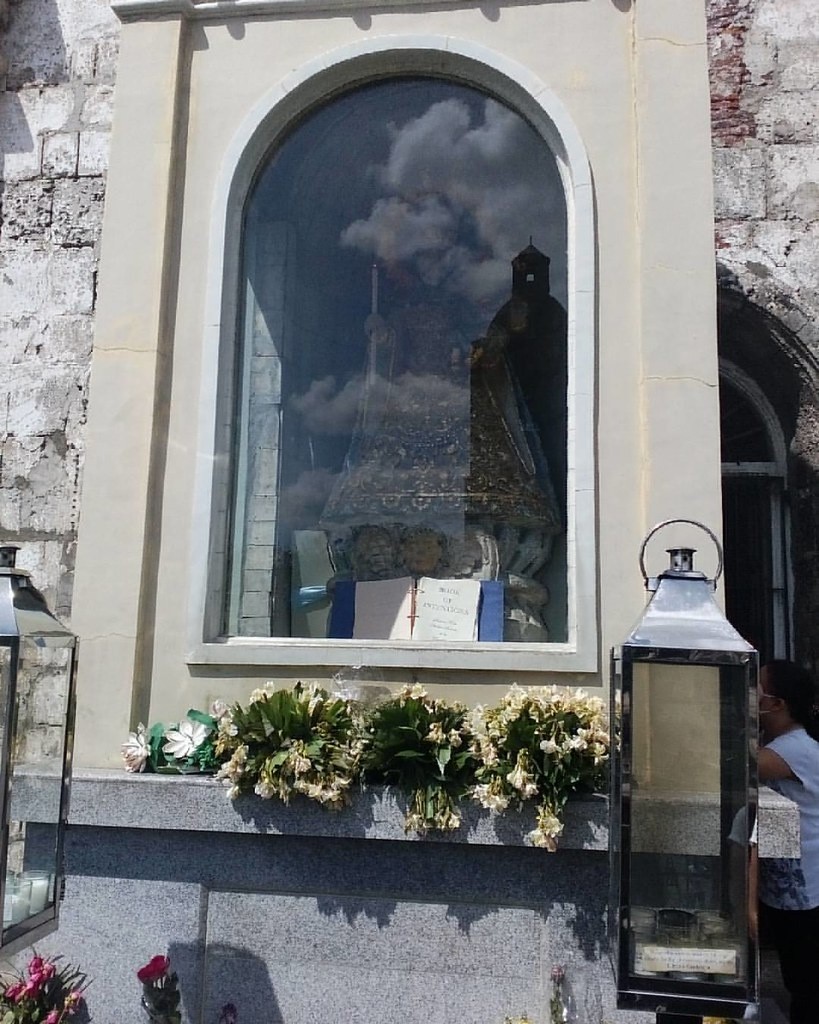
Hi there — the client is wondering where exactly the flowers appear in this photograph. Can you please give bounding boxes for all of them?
[505,963,570,1024]
[213,681,369,808]
[357,681,490,838]
[122,708,225,776]
[470,681,623,848]
[133,953,186,1024]
[218,1003,240,1024]
[0,954,86,1024]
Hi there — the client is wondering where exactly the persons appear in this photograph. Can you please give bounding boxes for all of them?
[756,661,819,1024]
[728,805,746,973]
